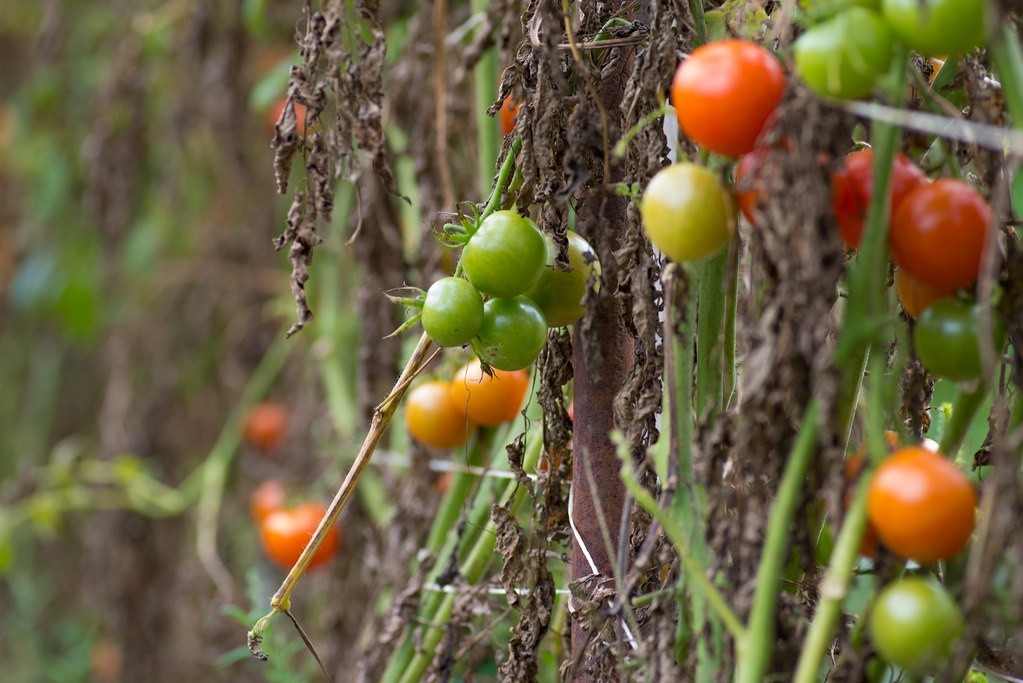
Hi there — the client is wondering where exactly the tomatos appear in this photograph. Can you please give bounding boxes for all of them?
[245,0,999,675]
